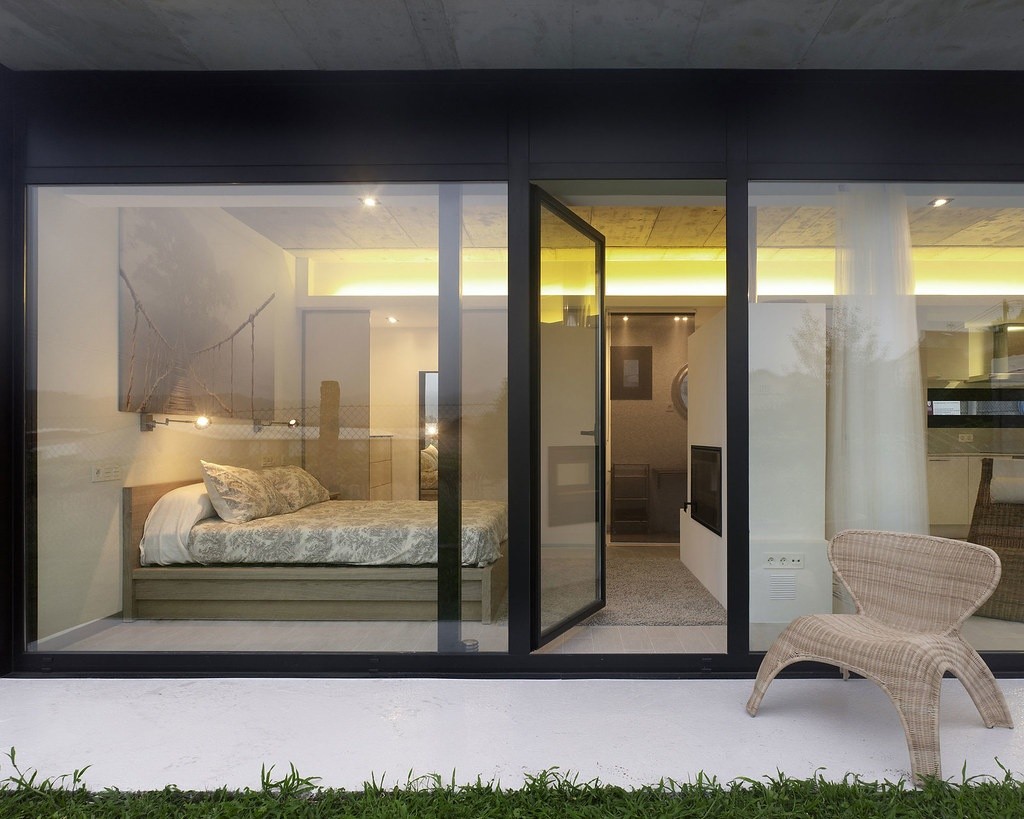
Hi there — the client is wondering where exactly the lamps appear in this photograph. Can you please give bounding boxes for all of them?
[140,414,211,432]
[253,419,300,433]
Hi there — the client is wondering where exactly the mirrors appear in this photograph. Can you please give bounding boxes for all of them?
[419,370,439,502]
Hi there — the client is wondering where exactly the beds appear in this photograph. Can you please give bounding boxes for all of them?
[419,471,438,502]
[122,478,508,625]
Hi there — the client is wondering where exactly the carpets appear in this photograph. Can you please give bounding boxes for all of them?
[494,556,729,627]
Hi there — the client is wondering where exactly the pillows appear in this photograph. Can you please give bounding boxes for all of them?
[200,460,294,524]
[419,444,438,472]
[258,465,331,513]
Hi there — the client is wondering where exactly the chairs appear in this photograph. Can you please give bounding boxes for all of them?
[746,529,1017,788]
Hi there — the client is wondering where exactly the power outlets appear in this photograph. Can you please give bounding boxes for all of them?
[761,553,803,570]
[92,464,121,482]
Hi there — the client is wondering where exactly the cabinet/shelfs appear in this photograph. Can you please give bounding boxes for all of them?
[367,435,393,503]
[612,463,653,543]
[655,468,688,543]
